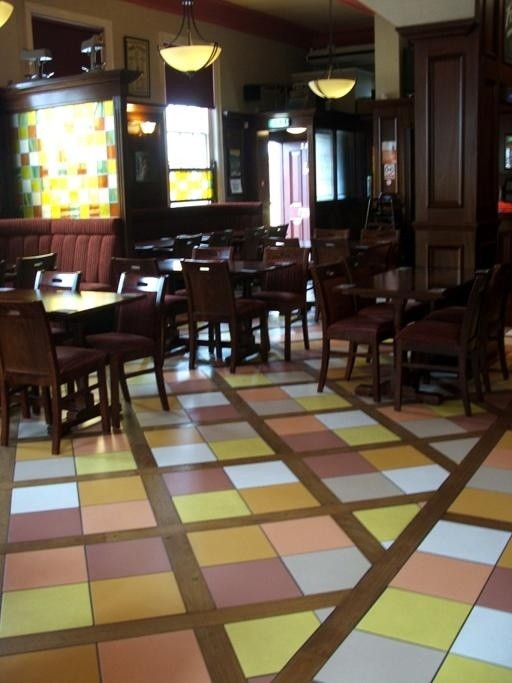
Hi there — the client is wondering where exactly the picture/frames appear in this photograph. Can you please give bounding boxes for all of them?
[124,36,150,98]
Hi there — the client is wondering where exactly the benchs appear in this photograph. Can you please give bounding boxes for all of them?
[129,202,262,239]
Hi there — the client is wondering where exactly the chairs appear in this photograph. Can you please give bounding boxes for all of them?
[310,223,403,257]
[2,223,171,455]
[308,256,510,419]
[171,224,313,374]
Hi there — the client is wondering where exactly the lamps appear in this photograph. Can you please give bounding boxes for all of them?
[136,121,156,149]
[308,0,357,100]
[157,0,223,80]
